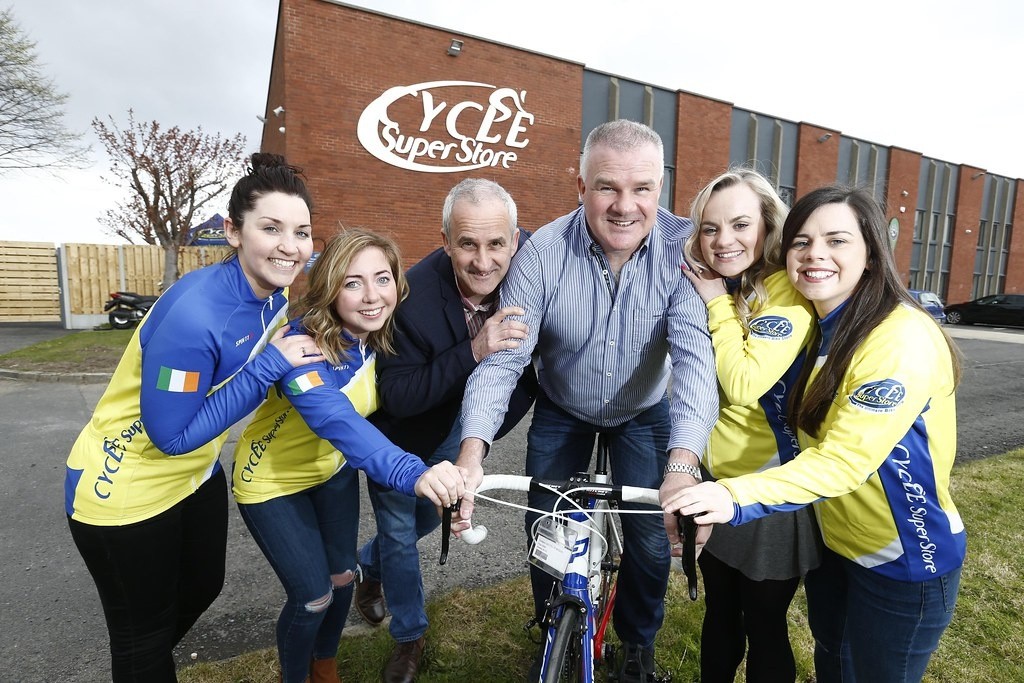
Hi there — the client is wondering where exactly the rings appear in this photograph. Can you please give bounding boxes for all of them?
[301,347,306,355]
[698,269,703,274]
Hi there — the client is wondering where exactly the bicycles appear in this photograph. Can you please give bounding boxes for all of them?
[437,471,697,683]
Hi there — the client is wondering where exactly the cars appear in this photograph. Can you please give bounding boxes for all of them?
[943,294,1024,329]
[900,289,947,326]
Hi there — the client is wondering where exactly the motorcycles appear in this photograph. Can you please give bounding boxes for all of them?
[104,290,164,329]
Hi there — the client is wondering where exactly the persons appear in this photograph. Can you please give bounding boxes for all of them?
[64,151,327,683]
[439,119,719,683]
[232,228,466,683]
[679,165,819,683]
[355,178,539,683]
[663,184,967,683]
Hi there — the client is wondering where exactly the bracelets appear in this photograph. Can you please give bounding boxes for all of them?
[664,464,702,483]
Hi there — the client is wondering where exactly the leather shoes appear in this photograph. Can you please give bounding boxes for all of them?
[353,548,387,625]
[382,636,426,683]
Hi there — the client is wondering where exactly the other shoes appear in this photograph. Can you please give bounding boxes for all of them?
[614,639,658,683]
[310,652,343,683]
